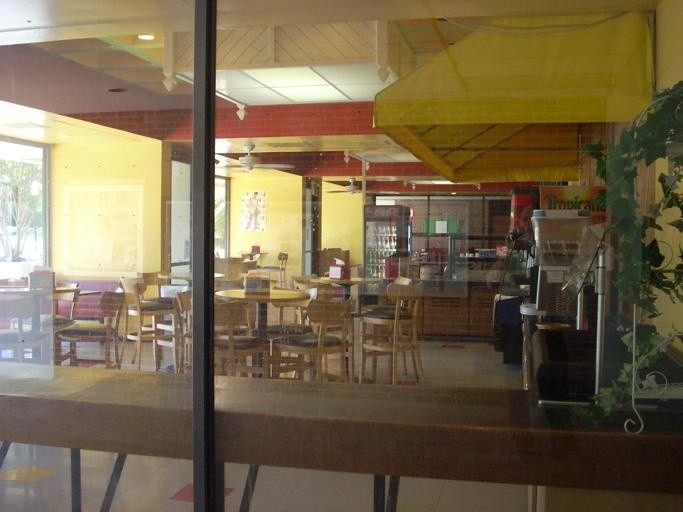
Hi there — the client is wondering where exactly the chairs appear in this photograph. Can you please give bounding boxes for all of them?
[211,298,268,379]
[260,254,291,290]
[266,299,314,335]
[63,294,125,369]
[133,282,174,372]
[252,247,262,262]
[360,279,422,387]
[53,288,79,367]
[268,296,352,383]
[174,288,198,376]
[143,271,176,304]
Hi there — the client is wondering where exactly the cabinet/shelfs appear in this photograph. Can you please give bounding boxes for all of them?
[449,233,514,284]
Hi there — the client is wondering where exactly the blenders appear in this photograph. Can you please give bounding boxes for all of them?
[517,207,580,322]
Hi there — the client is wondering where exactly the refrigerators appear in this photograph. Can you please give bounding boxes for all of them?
[363,205,410,305]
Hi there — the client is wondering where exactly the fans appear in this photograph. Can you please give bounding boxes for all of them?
[214,138,296,172]
[326,177,378,196]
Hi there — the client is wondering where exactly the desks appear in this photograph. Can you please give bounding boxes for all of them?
[519,300,578,392]
